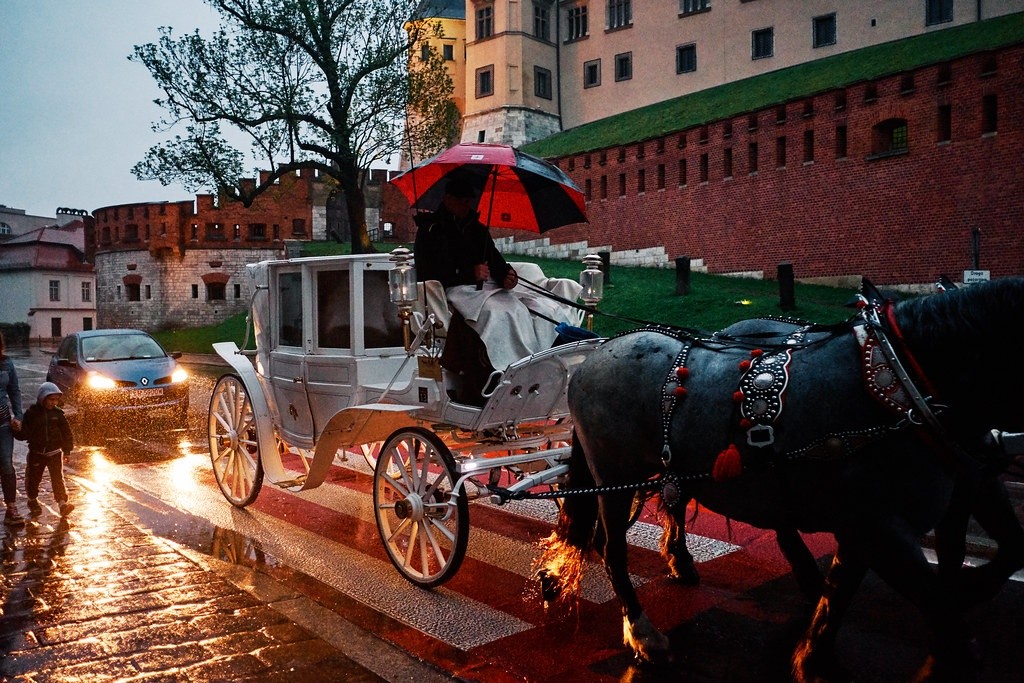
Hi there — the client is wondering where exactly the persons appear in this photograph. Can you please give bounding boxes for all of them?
[414,166,518,406]
[11,382,75,516]
[0,332,25,526]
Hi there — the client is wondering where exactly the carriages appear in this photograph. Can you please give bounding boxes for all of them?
[206,246,1024,682]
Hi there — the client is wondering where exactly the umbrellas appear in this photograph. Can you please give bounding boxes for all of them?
[391,141,591,290]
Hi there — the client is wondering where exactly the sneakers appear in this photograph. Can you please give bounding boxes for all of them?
[27,499,42,515]
[4,506,25,525]
[60,504,74,516]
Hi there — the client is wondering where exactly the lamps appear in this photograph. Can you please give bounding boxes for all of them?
[388,244,419,350]
[580,250,603,332]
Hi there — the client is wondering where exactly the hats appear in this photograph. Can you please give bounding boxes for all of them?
[445,176,480,198]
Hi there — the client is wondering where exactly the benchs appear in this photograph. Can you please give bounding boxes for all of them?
[406,261,583,350]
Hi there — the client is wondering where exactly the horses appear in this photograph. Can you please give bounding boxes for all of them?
[528,276,1024,676]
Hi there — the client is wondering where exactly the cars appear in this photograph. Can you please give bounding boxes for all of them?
[46,328,190,422]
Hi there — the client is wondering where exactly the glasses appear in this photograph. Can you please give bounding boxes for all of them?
[464,198,479,204]
